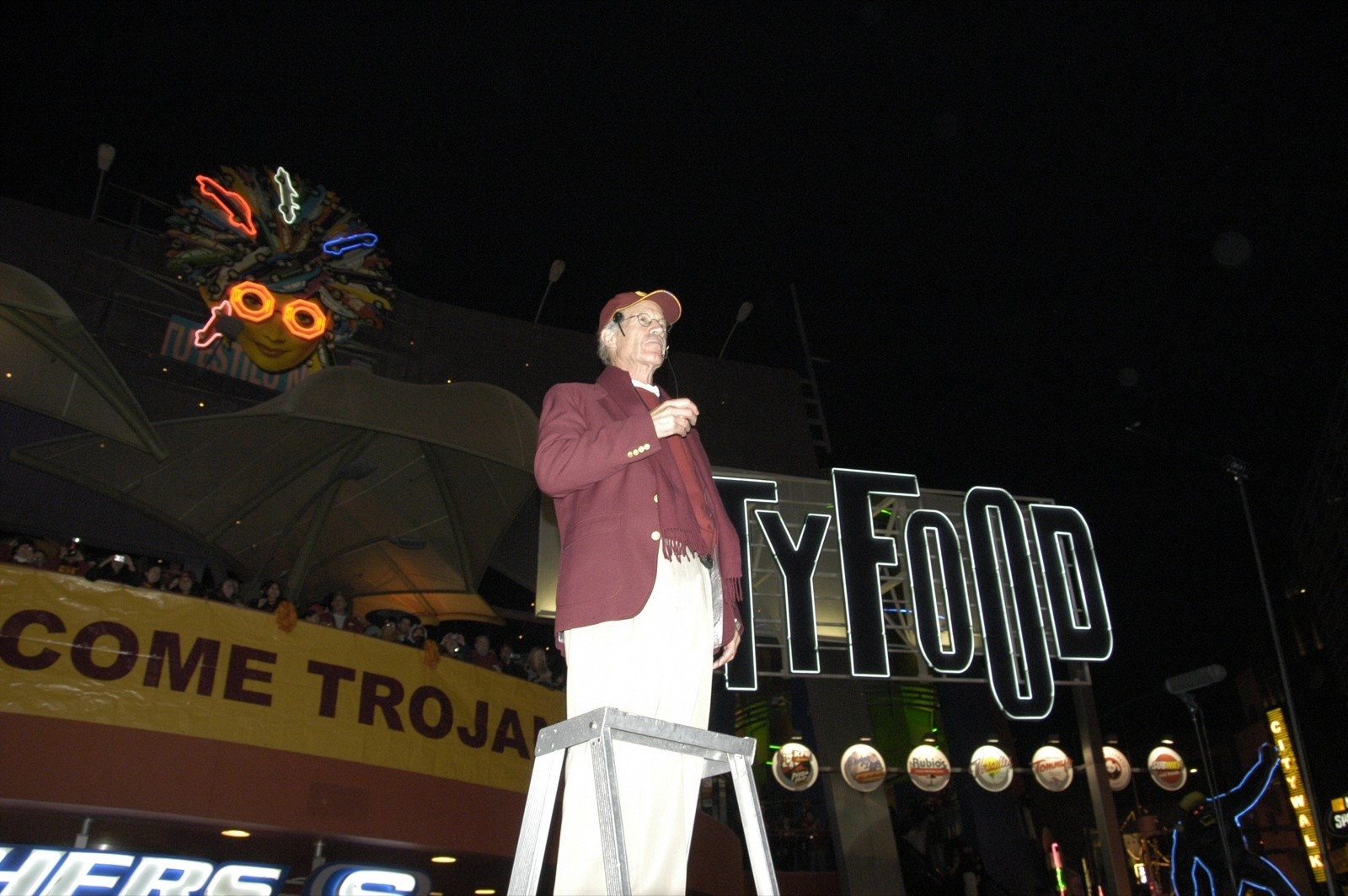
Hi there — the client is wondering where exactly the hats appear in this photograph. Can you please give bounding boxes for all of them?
[597,289,682,335]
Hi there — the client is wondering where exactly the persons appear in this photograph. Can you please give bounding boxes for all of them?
[3,534,570,689]
[168,162,399,373]
[535,289,745,896]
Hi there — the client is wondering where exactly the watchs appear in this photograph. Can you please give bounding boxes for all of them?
[735,618,744,636]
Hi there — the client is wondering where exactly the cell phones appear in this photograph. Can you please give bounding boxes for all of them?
[114,555,125,562]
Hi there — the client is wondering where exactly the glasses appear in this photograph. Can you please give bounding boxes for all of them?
[609,314,673,333]
